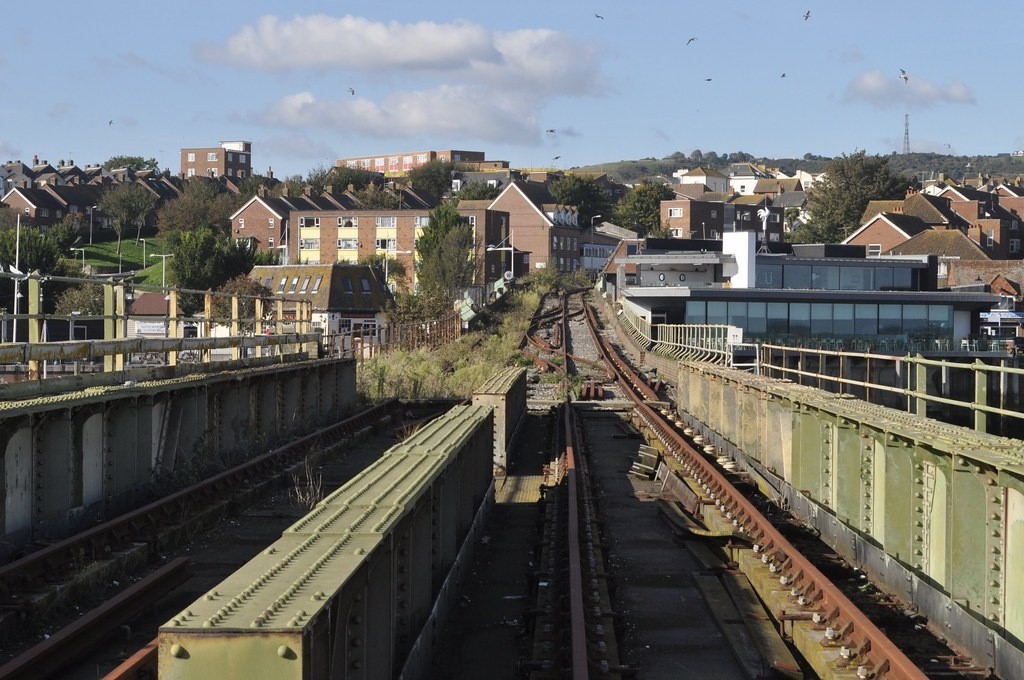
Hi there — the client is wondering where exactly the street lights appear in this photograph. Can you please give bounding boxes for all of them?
[591,215,602,268]
[385,249,413,282]
[702,222,705,240]
[277,245,289,266]
[150,254,174,293]
[741,213,749,231]
[70,248,85,273]
[487,248,514,278]
[998,313,1001,335]
[90,205,97,245]
[140,238,145,269]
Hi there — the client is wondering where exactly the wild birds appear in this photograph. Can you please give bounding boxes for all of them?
[553,156,561,160]
[705,79,713,82]
[594,13,605,20]
[545,128,557,134]
[898,68,910,85]
[346,87,355,95]
[803,10,812,21]
[781,73,787,78]
[108,119,114,127]
[686,37,698,46]
